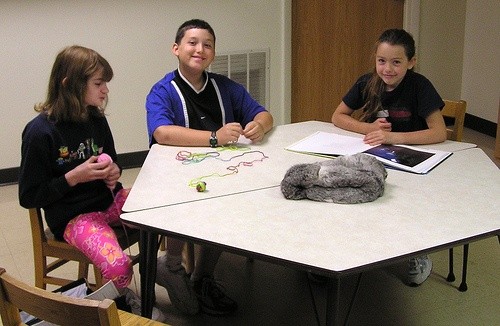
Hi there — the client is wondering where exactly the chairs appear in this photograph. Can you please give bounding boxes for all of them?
[433,98,469,141]
[28,194,144,292]
[0,267,171,326]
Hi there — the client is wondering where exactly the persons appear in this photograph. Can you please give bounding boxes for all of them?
[145,19,273,316]
[331,28,447,287]
[18,45,200,322]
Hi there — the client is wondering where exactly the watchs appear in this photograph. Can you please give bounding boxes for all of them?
[209,132,217,148]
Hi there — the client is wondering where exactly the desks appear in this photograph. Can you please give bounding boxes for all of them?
[119,120,500,326]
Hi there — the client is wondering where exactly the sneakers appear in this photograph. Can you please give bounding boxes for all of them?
[407,254,433,287]
[119,287,165,322]
[155,254,200,315]
[186,271,234,316]
